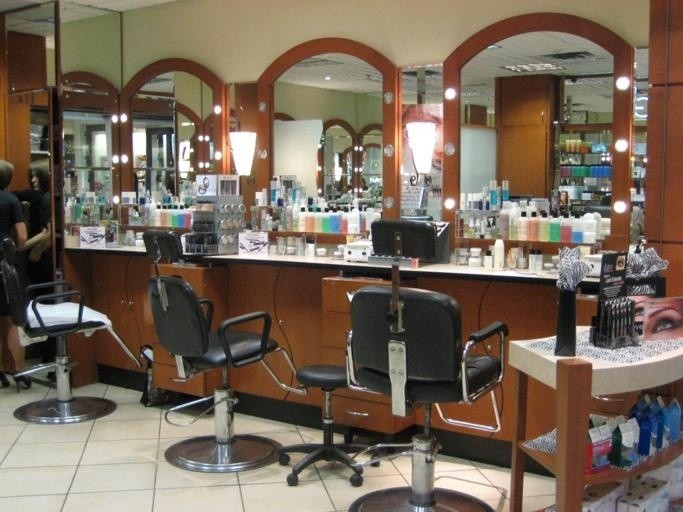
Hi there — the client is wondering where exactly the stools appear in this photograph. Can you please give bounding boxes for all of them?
[277,365,381,486]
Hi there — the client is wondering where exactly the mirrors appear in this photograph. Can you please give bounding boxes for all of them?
[57,3,120,246]
[442,9,634,258]
[255,33,397,250]
[121,55,226,244]
[2,0,59,390]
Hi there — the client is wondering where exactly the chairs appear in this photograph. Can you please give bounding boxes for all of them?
[345,265,509,512]
[0,237,141,426]
[149,262,307,474]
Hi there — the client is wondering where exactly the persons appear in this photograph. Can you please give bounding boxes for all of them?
[626,295,655,338]
[0,161,48,392]
[645,298,682,339]
[561,191,568,205]
[27,160,56,381]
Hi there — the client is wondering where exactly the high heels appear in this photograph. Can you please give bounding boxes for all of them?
[0,371,9,388]
[14,371,31,393]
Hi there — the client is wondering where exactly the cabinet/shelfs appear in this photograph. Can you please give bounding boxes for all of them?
[509,325,683,512]
